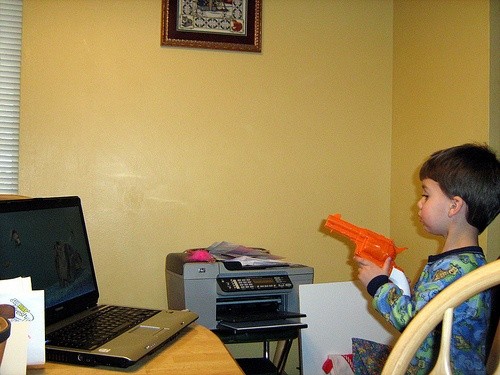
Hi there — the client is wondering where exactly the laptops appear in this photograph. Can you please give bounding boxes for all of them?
[0,195,199,369]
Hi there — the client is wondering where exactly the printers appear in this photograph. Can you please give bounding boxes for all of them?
[165,252,314,333]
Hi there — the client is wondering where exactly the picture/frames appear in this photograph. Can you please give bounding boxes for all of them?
[160,0,263,53]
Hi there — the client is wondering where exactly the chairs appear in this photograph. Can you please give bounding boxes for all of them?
[375,255,500,375]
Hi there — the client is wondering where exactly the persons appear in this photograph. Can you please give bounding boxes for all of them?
[352,142,500,375]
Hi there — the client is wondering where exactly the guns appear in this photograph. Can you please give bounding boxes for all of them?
[325,214,411,298]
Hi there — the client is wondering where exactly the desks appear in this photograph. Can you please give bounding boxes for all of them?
[26,321,246,374]
